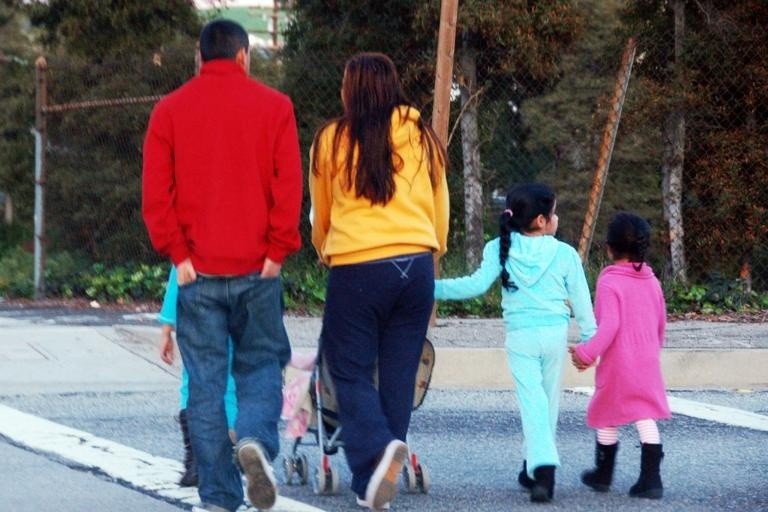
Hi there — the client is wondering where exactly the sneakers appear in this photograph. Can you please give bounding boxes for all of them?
[356,439,409,510]
[235,440,278,511]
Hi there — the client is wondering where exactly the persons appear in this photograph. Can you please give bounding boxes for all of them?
[308,52,449,510]
[434,184,600,503]
[141,19,303,510]
[158,260,243,486]
[567,212,671,500]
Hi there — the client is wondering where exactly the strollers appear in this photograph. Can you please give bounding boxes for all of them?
[279,336,437,496]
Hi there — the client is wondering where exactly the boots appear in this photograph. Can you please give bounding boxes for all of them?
[581,440,620,492]
[630,441,664,499]
[178,409,196,486]
[518,460,555,502]
